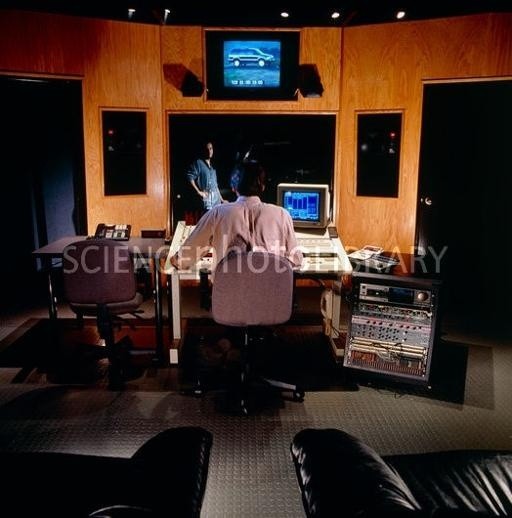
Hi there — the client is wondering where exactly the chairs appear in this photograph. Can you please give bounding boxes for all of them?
[91,222,133,243]
[58,238,144,382]
[194,251,306,418]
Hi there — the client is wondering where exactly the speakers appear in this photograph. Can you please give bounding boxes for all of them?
[183,76,203,96]
[299,64,321,96]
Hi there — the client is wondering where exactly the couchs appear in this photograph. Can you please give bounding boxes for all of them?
[289,427,512,518]
[0,426,213,518]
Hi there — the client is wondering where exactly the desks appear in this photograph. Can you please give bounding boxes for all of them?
[32,234,171,367]
[163,219,353,364]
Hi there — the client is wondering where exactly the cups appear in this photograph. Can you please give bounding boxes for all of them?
[165,235,172,245]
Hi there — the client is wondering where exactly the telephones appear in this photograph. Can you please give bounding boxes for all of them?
[86,224,131,242]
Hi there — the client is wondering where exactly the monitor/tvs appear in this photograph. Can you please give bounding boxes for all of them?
[208,33,298,102]
[275,182,323,229]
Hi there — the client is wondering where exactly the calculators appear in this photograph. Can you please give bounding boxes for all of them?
[348,245,384,261]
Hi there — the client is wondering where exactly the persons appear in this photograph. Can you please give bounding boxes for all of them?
[185,142,230,215]
[171,159,303,288]
